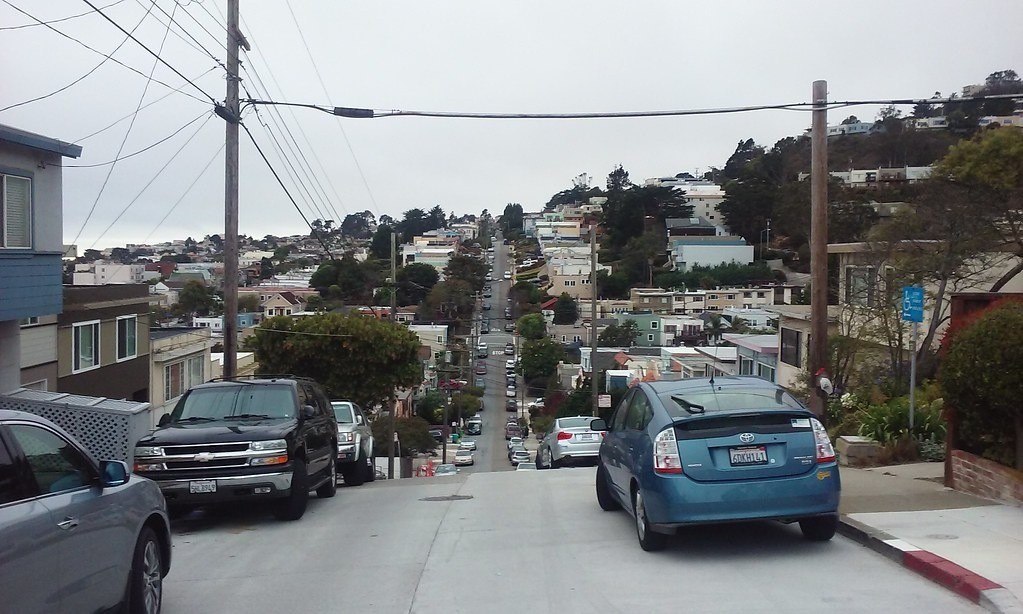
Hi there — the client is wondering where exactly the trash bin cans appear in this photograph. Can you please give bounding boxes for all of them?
[459,430,464,437]
[521,426,528,436]
[451,434,459,443]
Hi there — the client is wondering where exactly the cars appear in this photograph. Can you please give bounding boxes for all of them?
[458,437,478,451]
[513,462,537,472]
[480,236,498,334]
[535,415,610,469]
[466,341,488,436]
[0,408,173,614]
[506,399,518,412]
[504,415,531,466]
[503,270,512,280]
[504,306,515,332]
[432,464,461,478]
[454,450,474,466]
[503,342,518,397]
[588,363,842,552]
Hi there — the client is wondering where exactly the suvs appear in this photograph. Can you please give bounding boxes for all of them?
[331,401,376,486]
[527,398,546,409]
[131,373,340,520]
